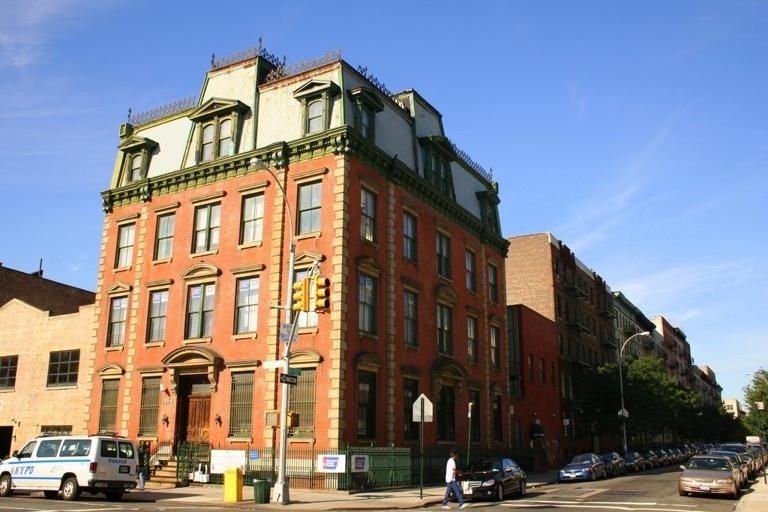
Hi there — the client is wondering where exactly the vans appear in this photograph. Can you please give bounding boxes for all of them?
[0,431,139,502]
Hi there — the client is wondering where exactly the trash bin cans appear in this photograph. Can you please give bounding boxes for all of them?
[225,467,270,503]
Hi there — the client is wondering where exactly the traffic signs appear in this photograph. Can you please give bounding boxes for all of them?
[263,360,302,385]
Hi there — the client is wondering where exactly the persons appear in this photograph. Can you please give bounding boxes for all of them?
[442,451,469,509]
[137,449,144,491]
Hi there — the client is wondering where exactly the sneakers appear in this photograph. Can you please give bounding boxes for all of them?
[459,503,469,508]
[442,505,451,509]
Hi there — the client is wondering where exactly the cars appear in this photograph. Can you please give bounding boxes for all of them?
[452,456,527,501]
[558,441,767,498]
[618,331,651,452]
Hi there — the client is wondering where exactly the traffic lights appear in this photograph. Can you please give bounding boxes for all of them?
[287,412,300,428]
[315,274,331,314]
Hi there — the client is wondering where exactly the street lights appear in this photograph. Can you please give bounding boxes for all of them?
[249,157,294,505]
